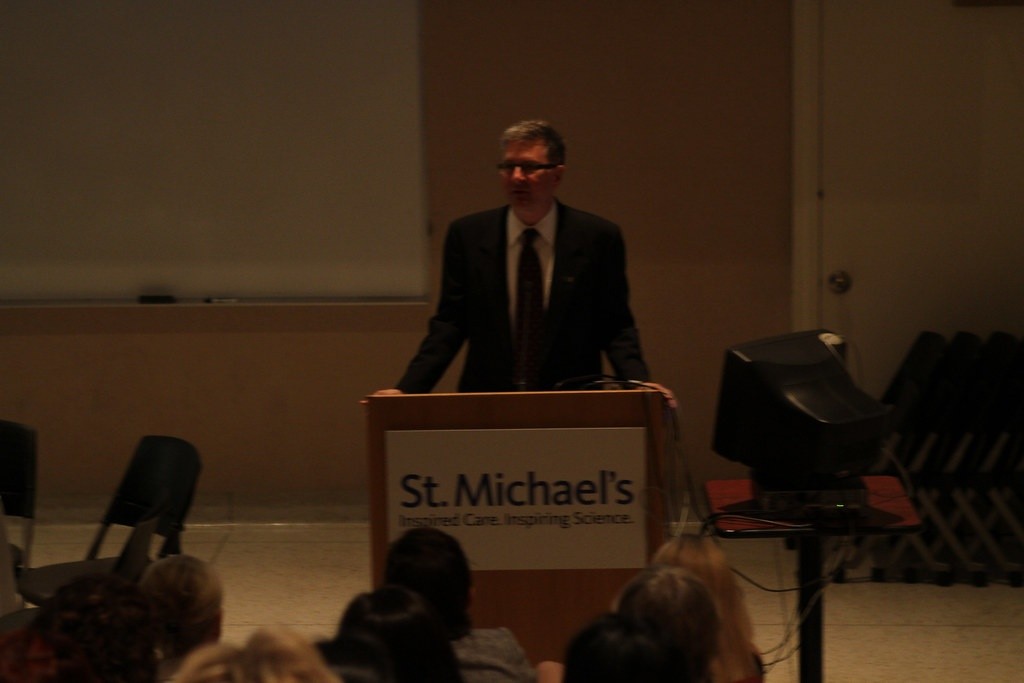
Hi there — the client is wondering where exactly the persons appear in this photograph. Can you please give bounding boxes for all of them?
[539,535,765,683]
[361,121,678,412]
[0,527,538,683]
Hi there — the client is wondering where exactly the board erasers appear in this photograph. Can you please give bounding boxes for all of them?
[137,295,178,304]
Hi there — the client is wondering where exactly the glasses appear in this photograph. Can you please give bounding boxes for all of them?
[496,158,559,174]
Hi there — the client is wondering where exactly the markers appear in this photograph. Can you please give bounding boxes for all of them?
[206,296,240,303]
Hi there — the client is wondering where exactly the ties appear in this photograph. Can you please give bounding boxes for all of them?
[514,228,545,386]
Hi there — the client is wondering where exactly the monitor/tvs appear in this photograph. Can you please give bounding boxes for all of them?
[711,328,884,493]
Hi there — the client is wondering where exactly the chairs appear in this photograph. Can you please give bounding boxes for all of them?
[27,433,205,604]
[0,419,39,575]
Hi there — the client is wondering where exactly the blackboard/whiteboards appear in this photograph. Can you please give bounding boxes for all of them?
[791,1,1023,390]
[0,3,431,308]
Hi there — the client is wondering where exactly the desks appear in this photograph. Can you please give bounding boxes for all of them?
[711,522,925,683]
